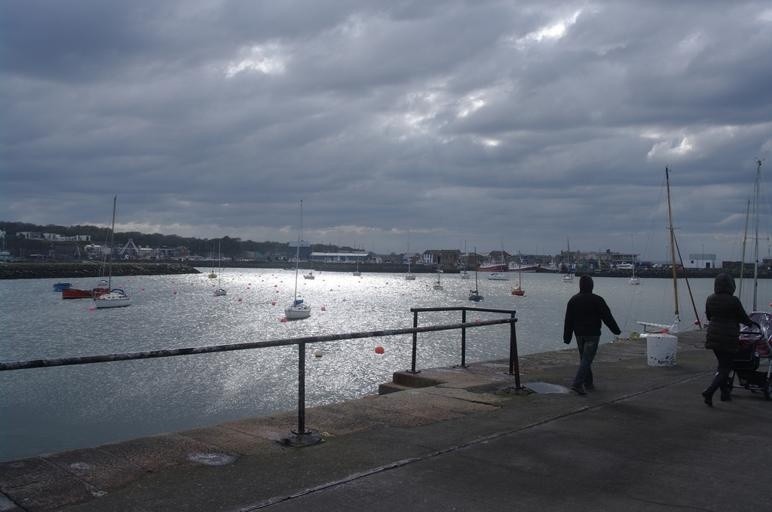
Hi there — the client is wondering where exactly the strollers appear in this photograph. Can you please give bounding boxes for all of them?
[732,311,772,401]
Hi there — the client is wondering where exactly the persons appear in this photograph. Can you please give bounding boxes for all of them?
[701,272,754,410]
[562,275,621,396]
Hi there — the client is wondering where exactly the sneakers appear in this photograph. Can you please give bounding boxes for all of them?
[702,391,712,406]
[720,396,731,401]
[572,384,593,395]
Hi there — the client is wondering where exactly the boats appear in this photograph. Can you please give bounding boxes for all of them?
[285,302,311,320]
[511,285,525,296]
[52,279,131,308]
[469,290,484,302]
